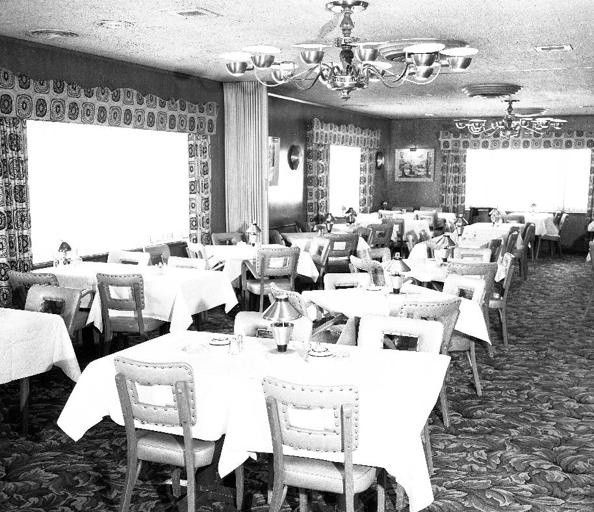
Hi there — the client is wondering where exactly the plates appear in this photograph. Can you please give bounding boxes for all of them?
[210,339,231,345]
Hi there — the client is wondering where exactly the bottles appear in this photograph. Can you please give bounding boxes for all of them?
[229,334,244,354]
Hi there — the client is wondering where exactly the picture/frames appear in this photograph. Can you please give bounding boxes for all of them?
[267,136,279,186]
[395,149,434,182]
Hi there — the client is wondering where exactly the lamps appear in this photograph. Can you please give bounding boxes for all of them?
[457,98,565,139]
[461,84,523,98]
[227,0,479,103]
